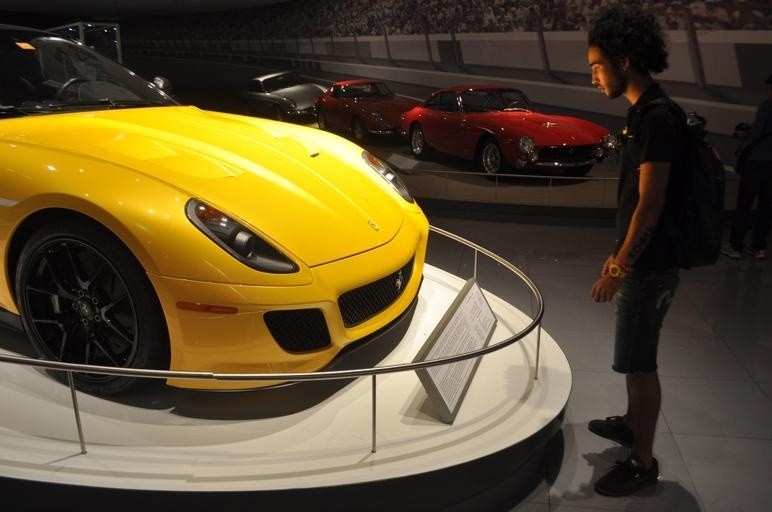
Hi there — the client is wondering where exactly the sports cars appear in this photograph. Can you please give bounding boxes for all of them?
[390,81,620,186]
[234,65,329,122]
[2,27,437,405]
[314,76,417,146]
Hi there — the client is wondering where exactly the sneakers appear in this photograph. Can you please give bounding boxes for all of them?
[587,415,636,449]
[744,244,766,259]
[594,453,658,498]
[719,243,741,259]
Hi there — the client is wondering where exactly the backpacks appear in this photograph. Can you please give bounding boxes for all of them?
[629,98,723,273]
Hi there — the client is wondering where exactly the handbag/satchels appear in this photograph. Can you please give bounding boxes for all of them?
[733,141,754,178]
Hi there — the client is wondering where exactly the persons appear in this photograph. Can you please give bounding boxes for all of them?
[583,4,691,498]
[718,73,772,260]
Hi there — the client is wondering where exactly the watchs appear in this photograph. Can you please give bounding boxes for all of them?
[608,263,628,279]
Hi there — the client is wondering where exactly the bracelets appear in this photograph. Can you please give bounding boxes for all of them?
[614,257,632,274]
